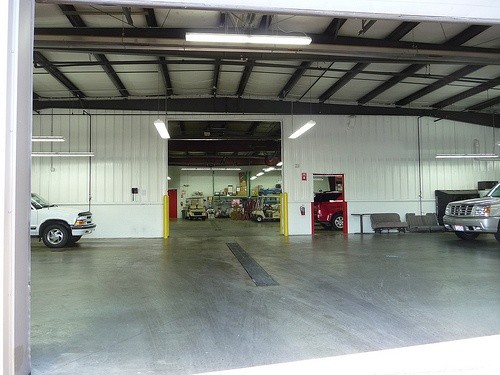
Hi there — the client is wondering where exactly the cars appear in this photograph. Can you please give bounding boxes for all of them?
[30,193,97,250]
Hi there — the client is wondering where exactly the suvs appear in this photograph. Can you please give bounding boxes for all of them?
[313,190,345,231]
[442,183,500,242]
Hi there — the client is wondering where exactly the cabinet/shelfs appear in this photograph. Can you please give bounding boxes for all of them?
[211,193,280,214]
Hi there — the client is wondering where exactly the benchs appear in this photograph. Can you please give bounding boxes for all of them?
[371,213,408,234]
[405,213,446,233]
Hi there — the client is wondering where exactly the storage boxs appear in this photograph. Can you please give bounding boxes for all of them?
[219,186,281,211]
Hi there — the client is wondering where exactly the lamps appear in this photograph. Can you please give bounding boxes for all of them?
[185,12,312,45]
[287,67,317,140]
[435,114,499,160]
[31,151,94,157]
[153,69,171,139]
[32,112,66,142]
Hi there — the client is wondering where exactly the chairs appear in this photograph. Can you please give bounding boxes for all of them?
[191,200,203,210]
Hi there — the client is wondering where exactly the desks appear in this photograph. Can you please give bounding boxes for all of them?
[351,214,373,234]
